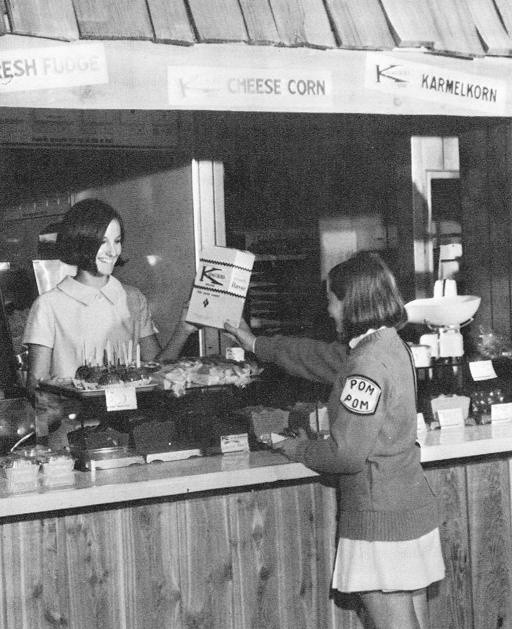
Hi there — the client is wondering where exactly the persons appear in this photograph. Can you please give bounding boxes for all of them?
[215,250,445,629]
[19,196,202,388]
